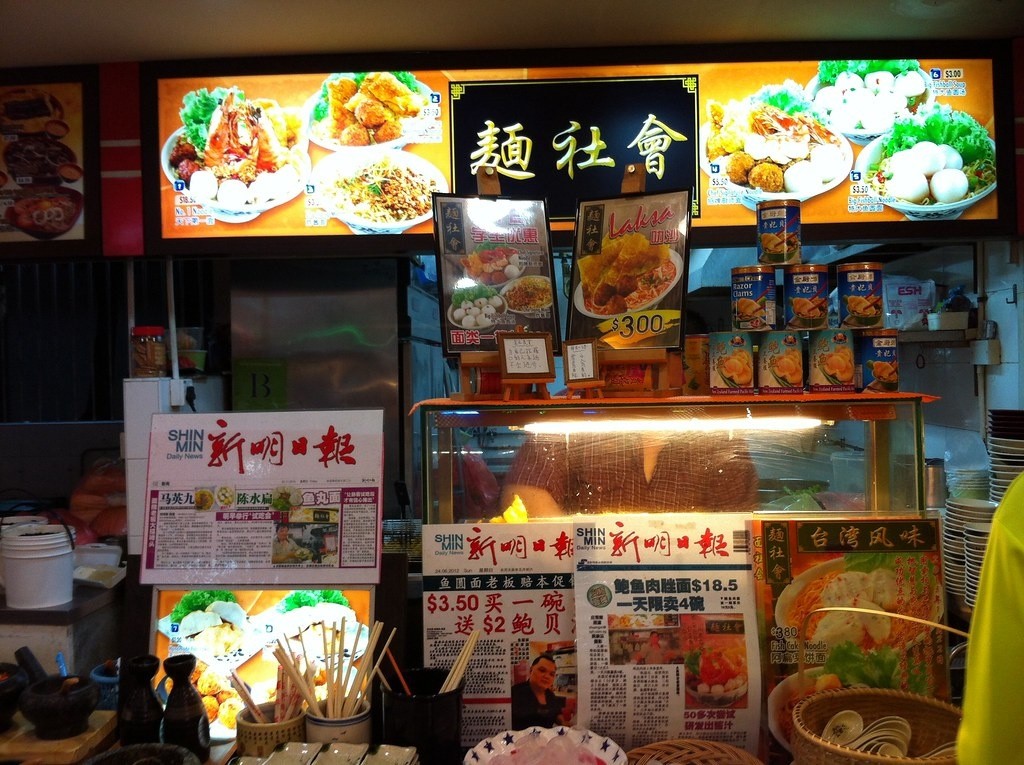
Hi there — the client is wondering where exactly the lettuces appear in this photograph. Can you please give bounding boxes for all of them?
[845,548,926,594]
[819,640,903,691]
[170,589,350,624]
[870,99,995,181]
[746,59,920,127]
[176,85,246,153]
[311,72,421,122]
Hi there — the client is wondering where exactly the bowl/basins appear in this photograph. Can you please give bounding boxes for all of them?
[0,663,25,733]
[269,492,304,509]
[500,275,553,318]
[295,548,309,559]
[160,124,313,223]
[772,556,944,658]
[446,293,509,334]
[308,146,450,234]
[19,673,102,740]
[300,81,436,152]
[82,744,201,765]
[0,88,83,238]
[463,259,527,289]
[686,680,747,707]
[168,349,206,372]
[162,326,204,350]
[925,311,969,331]
[698,67,996,221]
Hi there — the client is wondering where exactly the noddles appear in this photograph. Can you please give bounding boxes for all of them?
[787,569,842,640]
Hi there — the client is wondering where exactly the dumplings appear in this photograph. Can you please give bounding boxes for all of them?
[181,601,358,656]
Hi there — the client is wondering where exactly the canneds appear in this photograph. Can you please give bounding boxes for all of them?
[680,198,900,396]
[130,326,166,378]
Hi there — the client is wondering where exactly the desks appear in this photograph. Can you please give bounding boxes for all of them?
[0,554,153,679]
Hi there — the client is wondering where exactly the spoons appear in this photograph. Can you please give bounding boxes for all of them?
[820,708,959,760]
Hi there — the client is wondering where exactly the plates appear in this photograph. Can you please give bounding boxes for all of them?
[942,497,999,596]
[985,408,1024,502]
[943,467,989,499]
[462,726,628,765]
[573,247,684,320]
[963,523,993,610]
[158,614,251,666]
[250,604,369,671]
[194,486,233,511]
[237,741,421,765]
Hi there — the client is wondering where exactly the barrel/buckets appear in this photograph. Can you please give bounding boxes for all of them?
[0,487,76,610]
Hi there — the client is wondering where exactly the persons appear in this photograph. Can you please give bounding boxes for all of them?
[954,470,1024,765]
[511,653,559,729]
[271,525,309,563]
[495,430,760,514]
[628,630,668,665]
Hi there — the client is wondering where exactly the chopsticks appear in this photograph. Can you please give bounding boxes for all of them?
[386,627,481,695]
[229,617,397,722]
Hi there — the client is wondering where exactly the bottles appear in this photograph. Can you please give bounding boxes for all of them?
[163,653,210,763]
[119,654,163,747]
[131,327,167,378]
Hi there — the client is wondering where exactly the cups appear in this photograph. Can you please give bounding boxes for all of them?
[380,668,465,765]
[305,697,372,744]
[235,702,306,757]
